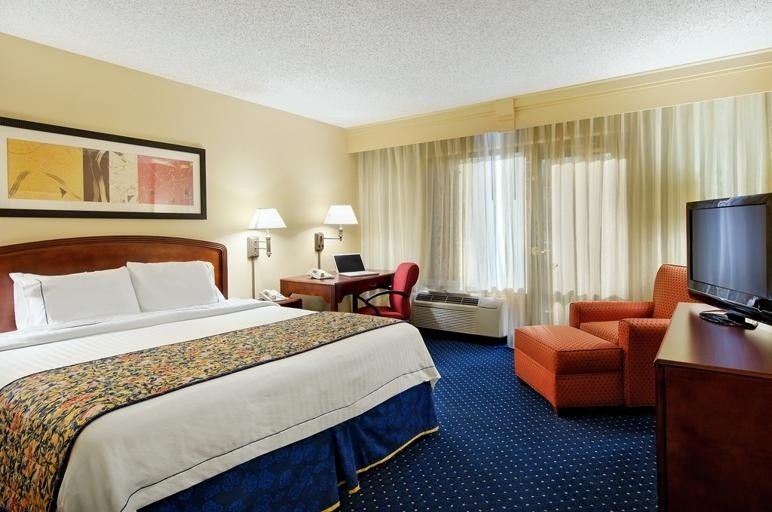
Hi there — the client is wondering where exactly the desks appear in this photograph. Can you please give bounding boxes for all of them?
[278,269,395,313]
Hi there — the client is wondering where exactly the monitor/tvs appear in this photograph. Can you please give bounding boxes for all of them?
[685,193,772,330]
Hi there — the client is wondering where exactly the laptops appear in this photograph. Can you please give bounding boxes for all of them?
[333,253,379,277]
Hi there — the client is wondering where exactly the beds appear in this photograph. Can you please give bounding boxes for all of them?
[0,233,442,512]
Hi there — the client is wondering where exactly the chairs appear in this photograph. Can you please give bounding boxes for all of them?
[354,261,419,321]
[568,264,708,418]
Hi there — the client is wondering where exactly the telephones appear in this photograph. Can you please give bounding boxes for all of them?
[259,289,289,301]
[308,268,335,280]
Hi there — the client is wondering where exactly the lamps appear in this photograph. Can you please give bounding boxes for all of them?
[312,204,358,269]
[246,206,288,259]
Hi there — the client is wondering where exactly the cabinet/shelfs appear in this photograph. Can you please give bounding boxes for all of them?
[652,301,771,512]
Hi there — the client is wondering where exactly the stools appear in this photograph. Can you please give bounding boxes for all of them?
[514,324,625,419]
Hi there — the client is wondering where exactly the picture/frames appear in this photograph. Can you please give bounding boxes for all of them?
[0,114,207,223]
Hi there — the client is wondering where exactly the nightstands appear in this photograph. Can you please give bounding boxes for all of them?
[275,296,302,309]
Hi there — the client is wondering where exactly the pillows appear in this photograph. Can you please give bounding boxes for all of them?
[124,258,225,314]
[5,266,141,332]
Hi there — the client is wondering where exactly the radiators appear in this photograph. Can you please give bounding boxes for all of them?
[410,290,508,346]
